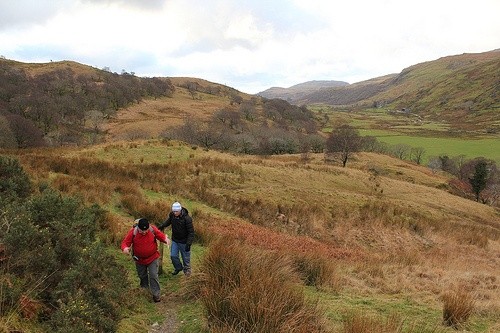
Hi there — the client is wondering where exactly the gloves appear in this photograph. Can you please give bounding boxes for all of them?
[184,244,191,252]
[158,224,164,231]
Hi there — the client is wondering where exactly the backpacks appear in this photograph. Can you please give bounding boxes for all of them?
[133,219,156,238]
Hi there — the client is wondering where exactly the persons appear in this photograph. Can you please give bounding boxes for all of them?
[121,218,172,302]
[156,201,194,276]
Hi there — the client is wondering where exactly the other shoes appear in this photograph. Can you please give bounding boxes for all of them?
[184,269,191,277]
[153,295,160,302]
[172,267,183,275]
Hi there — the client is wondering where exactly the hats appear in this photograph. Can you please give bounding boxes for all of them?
[172,201,182,211]
[138,218,149,230]
